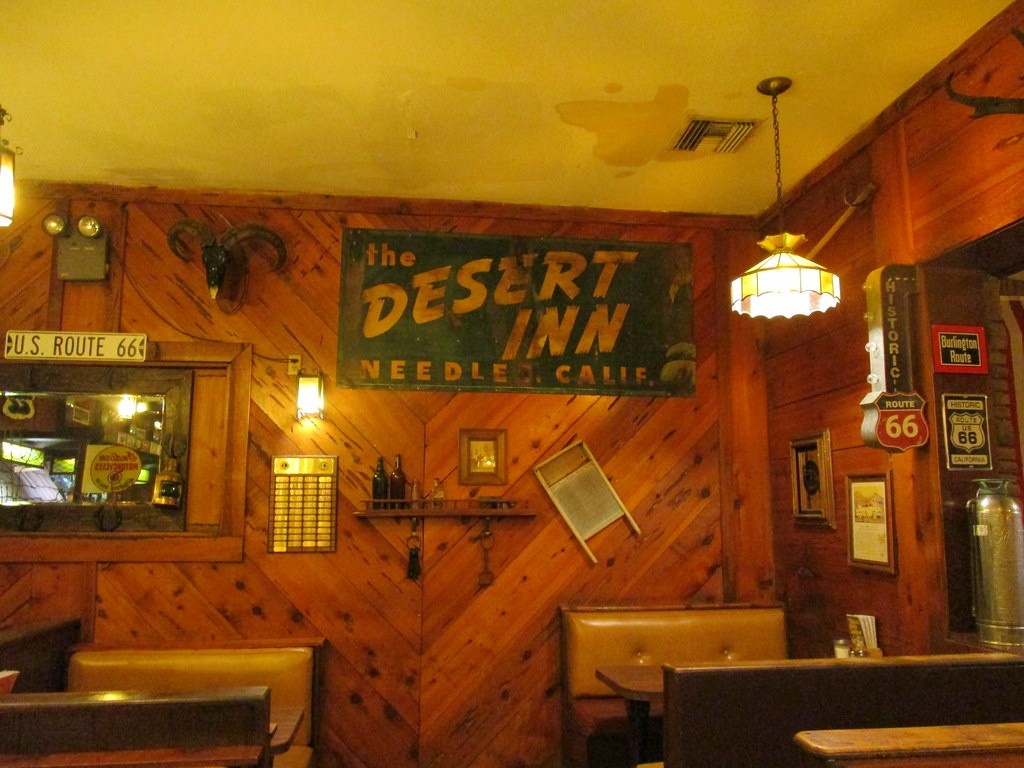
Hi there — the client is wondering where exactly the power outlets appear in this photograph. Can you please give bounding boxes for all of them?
[288,355,301,375]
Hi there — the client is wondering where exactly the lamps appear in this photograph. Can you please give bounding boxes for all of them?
[76,215,102,239]
[0,103,23,226]
[730,76,842,321]
[40,213,69,239]
[296,367,328,420]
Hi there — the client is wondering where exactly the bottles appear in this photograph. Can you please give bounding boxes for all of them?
[390,454,405,508]
[411,479,423,510]
[429,478,445,510]
[152,456,183,509]
[373,456,387,510]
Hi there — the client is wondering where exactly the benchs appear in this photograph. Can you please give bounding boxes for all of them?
[558,601,788,768]
[0,686,278,768]
[634,652,1024,768]
[792,717,1024,768]
[65,637,327,768]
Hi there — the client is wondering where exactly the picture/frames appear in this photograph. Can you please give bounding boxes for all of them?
[789,427,837,532]
[458,427,507,486]
[845,468,897,577]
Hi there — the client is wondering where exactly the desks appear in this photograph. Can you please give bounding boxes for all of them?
[0,744,266,768]
[269,702,305,755]
[594,666,663,768]
